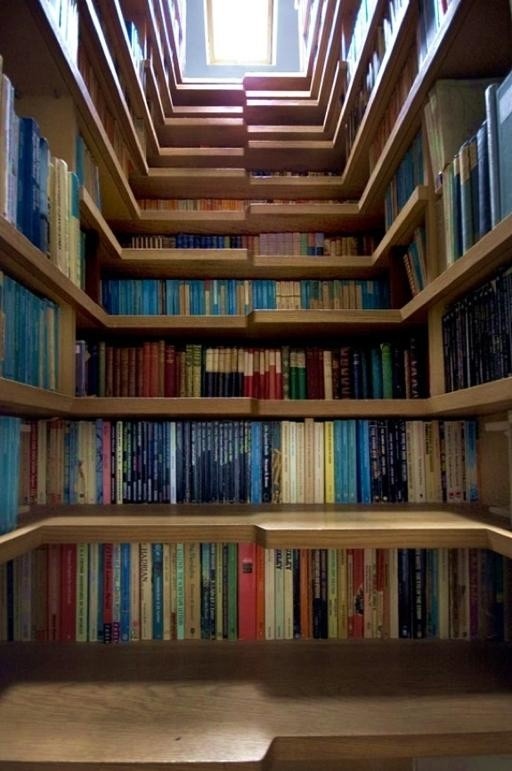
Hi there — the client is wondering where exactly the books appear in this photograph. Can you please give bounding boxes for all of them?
[1,415,512,537]
[42,0,455,211]
[0,60,511,400]
[1,543,512,641]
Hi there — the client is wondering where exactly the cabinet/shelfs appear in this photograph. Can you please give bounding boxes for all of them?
[1,332,510,416]
[2,545,512,771]
[0,413,510,559]
[1,44,512,329]
